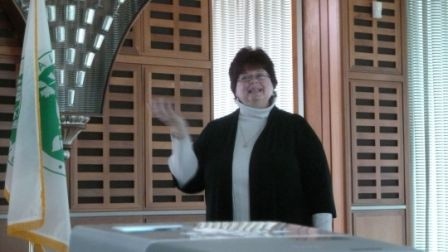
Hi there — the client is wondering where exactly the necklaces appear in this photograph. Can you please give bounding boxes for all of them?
[239,125,261,148]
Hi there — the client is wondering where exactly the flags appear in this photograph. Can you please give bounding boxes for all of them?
[4,0,72,252]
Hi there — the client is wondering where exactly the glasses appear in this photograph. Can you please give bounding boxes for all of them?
[238,72,271,82]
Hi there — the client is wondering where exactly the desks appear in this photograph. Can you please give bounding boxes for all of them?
[69,220,422,252]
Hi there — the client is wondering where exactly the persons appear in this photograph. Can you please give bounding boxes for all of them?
[152,47,337,232]
